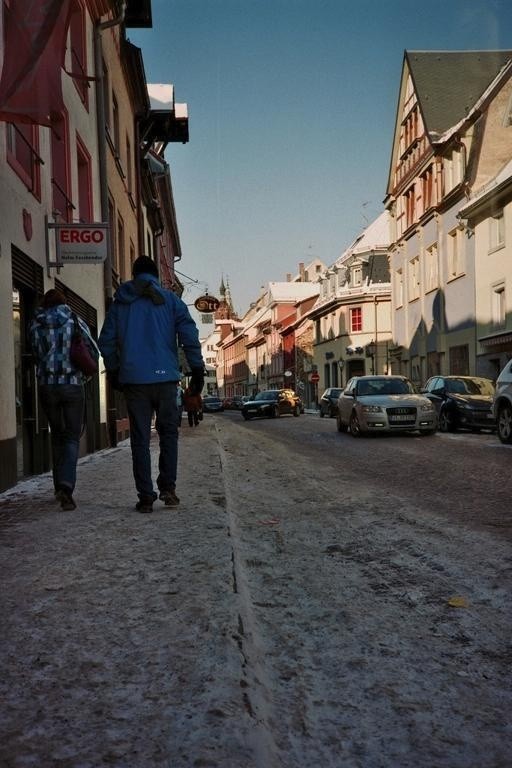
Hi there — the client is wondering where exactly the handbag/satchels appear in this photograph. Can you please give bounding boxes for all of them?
[69,333,99,375]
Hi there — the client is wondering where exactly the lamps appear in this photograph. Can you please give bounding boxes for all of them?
[326,348,364,360]
[281,370,293,377]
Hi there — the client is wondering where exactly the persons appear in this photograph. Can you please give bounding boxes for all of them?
[96,254,206,513]
[23,288,93,513]
[176,382,204,427]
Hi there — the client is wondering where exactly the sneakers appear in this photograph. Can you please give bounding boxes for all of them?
[136,501,153,513]
[159,490,179,505]
[55,488,76,511]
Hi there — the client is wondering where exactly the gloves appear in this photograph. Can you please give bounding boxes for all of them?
[188,366,205,396]
[107,368,126,391]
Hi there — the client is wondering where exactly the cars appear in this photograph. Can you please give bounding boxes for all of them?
[424,359,512,444]
[241,389,303,418]
[317,386,341,418]
[335,375,438,437]
[202,396,246,413]
